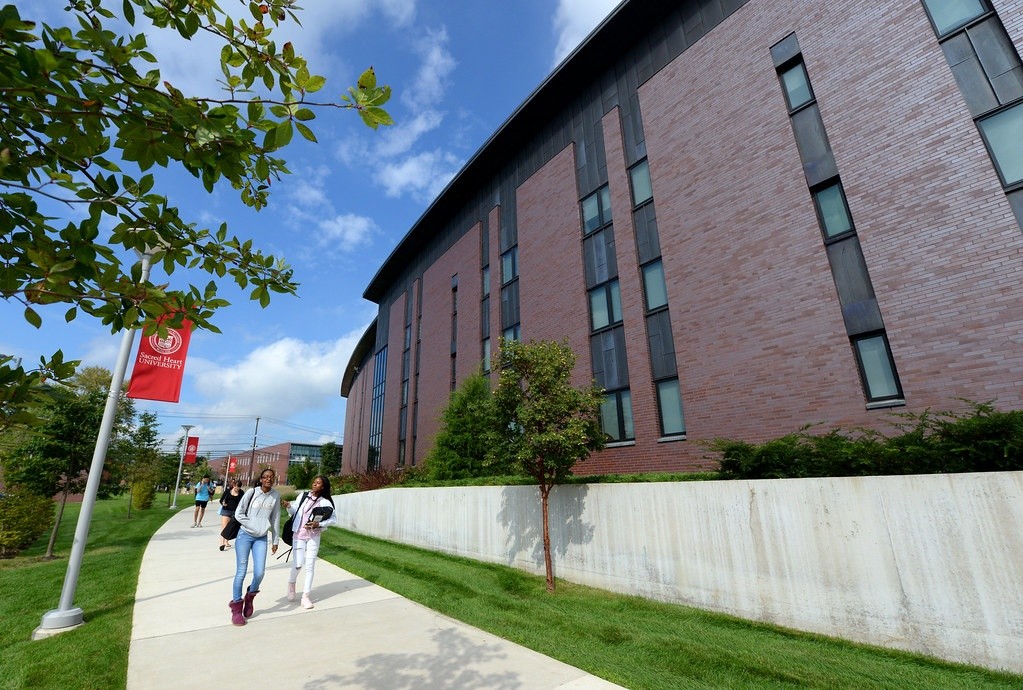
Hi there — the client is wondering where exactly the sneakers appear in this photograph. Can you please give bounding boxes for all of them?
[287,583,296,600]
[301,597,314,609]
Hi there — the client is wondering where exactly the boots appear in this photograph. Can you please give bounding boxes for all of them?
[228,599,245,626]
[243,586,260,617]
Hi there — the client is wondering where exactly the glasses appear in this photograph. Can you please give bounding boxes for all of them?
[261,475,276,480]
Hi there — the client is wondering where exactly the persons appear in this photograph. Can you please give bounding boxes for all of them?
[193,474,214,528]
[209,479,216,502]
[281,475,335,609]
[219,481,245,551]
[228,469,279,626]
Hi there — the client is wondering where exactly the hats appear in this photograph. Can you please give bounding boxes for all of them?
[204,474,209,478]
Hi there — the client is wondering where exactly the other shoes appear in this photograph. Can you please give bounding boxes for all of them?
[225,543,231,547]
[220,545,225,550]
[197,522,202,527]
[191,522,197,528]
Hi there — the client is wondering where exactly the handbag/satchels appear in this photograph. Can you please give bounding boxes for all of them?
[282,517,294,547]
[217,502,224,515]
[221,516,241,540]
